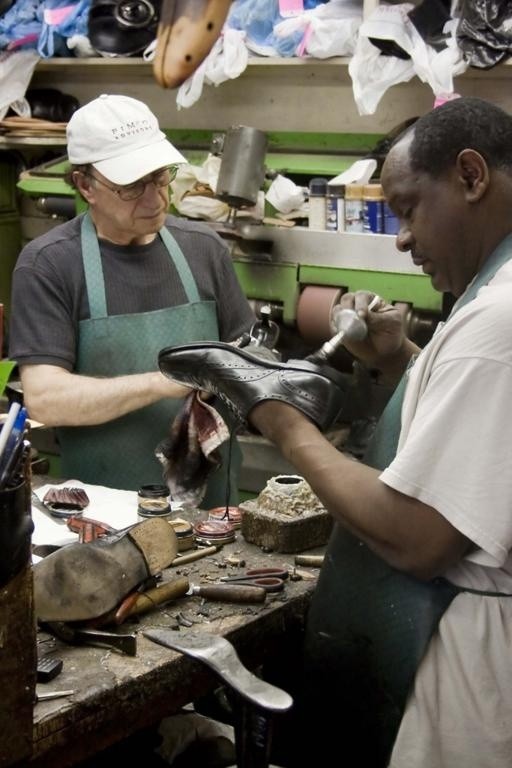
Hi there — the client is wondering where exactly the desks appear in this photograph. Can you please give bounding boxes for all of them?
[2,475,336,768]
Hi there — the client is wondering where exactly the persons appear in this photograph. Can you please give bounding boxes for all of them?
[9,93,262,725]
[247,96,511,767]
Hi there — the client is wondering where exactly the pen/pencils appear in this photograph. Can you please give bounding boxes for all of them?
[0,402,28,494]
[165,543,223,568]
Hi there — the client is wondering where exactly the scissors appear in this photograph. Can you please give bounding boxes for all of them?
[207,567,289,593]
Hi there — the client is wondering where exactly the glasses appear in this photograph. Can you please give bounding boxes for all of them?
[84,167,180,201]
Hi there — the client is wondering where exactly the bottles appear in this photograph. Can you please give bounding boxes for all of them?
[167,521,194,552]
[308,178,401,236]
[137,484,172,523]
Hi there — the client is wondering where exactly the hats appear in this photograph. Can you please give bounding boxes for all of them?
[66,93,188,186]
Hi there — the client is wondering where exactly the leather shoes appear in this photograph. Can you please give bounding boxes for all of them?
[158,341,348,434]
[32,517,179,622]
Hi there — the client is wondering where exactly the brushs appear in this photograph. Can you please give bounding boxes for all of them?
[304,293,381,362]
